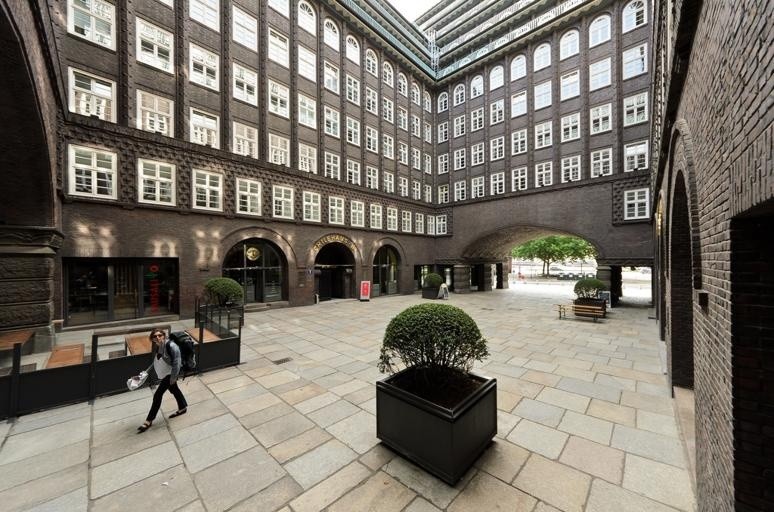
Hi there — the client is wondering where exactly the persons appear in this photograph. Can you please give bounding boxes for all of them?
[136,328,188,434]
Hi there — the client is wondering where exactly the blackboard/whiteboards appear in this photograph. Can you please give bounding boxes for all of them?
[442,285,450,298]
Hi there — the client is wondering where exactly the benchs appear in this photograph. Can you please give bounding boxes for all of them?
[552,303,605,322]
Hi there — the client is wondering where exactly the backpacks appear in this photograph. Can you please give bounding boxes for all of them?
[166,331,198,373]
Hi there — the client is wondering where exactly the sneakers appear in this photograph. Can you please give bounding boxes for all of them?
[137,419,152,432]
[173,407,186,416]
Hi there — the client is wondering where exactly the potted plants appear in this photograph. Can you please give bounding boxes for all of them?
[201,276,246,328]
[421,272,443,299]
[374,302,497,489]
[573,278,607,319]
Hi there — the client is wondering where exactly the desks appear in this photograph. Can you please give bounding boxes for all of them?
[0,329,36,374]
[44,343,85,369]
[125,334,156,356]
[185,327,221,345]
